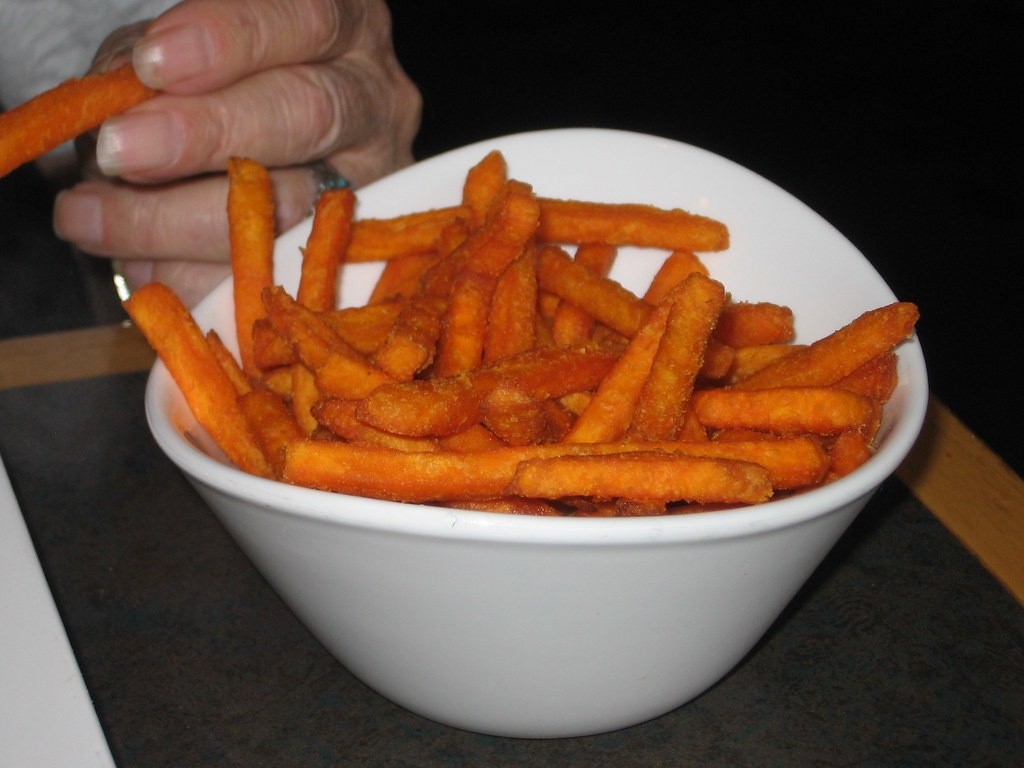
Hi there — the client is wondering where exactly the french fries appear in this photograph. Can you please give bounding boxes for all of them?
[0,61,162,181]
[122,148,919,518]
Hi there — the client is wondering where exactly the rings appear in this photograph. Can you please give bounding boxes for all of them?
[311,160,351,201]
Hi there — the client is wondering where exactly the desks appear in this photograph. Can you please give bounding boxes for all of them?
[1,318,1024,767]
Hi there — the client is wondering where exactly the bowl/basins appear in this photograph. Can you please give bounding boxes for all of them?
[144,128,930,741]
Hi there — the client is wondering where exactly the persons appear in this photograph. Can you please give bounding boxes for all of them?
[0,0,423,314]
